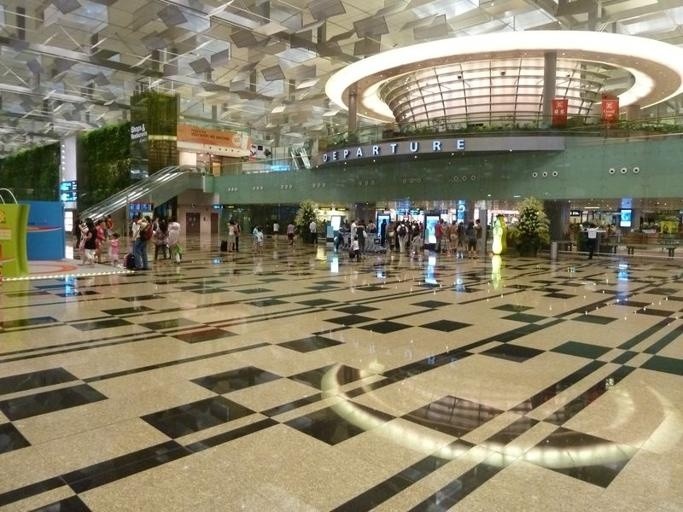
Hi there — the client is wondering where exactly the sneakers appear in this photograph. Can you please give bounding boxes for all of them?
[77,260,118,265]
[133,267,152,270]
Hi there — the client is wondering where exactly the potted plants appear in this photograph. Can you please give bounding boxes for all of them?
[507,196,550,258]
[294,199,320,244]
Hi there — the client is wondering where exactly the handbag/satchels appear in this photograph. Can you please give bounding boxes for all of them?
[176,243,186,255]
[123,253,135,269]
[349,247,355,259]
[450,233,458,240]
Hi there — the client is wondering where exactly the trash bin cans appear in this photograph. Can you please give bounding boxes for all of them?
[213,160,222,177]
[628,246,634,254]
[564,244,573,252]
[667,248,675,257]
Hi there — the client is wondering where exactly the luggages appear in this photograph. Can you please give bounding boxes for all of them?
[220,240,227,252]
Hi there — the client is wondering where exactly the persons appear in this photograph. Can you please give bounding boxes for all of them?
[332,217,424,261]
[286,221,295,246]
[309,221,320,247]
[225,219,242,253]
[74,210,183,271]
[273,220,280,234]
[584,223,598,260]
[433,217,483,253]
[252,224,266,253]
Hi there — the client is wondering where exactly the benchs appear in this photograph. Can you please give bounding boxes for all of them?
[626,243,677,257]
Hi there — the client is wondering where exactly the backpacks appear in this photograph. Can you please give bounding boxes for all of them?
[140,223,153,240]
[399,225,406,237]
